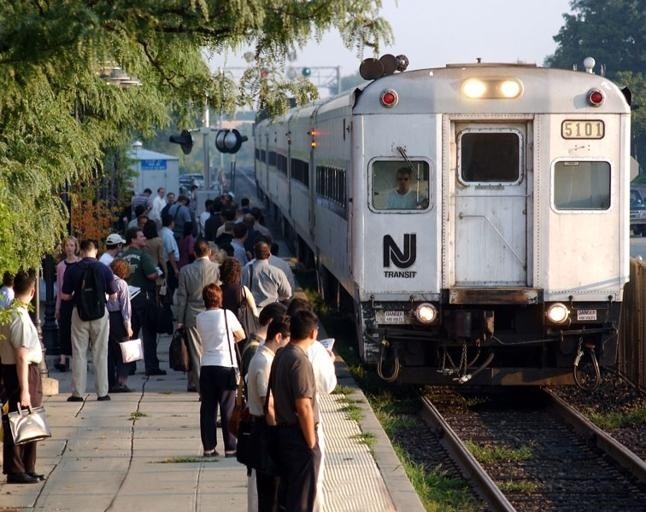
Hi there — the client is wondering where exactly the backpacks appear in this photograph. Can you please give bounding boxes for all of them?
[74,261,107,321]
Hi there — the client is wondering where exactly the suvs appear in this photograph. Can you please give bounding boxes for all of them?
[629,187,646,237]
[179,172,205,192]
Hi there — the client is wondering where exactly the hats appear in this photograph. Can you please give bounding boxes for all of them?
[105,233,127,246]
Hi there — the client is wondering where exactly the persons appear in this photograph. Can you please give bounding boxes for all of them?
[386,168,429,211]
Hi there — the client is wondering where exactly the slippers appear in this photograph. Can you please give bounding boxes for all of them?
[203,450,219,457]
[225,451,237,458]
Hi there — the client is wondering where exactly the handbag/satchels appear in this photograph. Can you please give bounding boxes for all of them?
[7,402,52,447]
[227,397,253,440]
[230,367,242,389]
[236,414,269,469]
[159,279,168,296]
[118,337,144,364]
[169,329,193,372]
[237,286,259,347]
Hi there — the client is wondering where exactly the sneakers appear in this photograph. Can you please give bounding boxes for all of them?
[108,384,131,393]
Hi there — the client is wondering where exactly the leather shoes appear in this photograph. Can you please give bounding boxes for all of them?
[97,395,110,401]
[6,473,45,484]
[67,397,84,402]
[145,368,167,376]
[58,363,66,373]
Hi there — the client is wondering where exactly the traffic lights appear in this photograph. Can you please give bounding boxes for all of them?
[302,67,310,75]
[168,130,191,155]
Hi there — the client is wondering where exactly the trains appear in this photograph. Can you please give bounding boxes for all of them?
[250,49,640,390]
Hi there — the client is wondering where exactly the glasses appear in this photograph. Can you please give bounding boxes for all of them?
[397,178,409,181]
